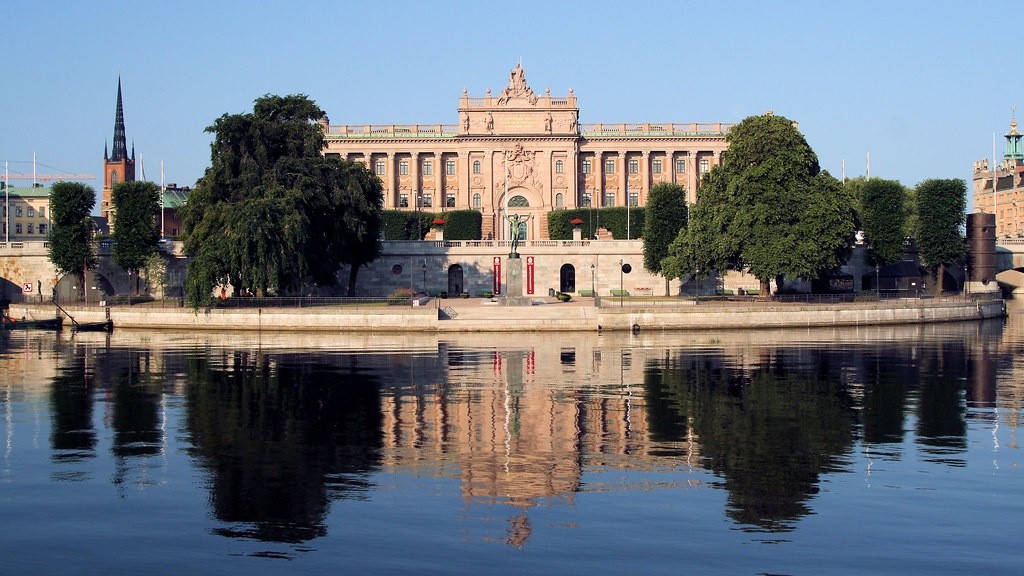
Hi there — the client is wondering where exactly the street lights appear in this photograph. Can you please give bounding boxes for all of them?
[874,264,880,301]
[422,262,427,290]
[964,263,968,302]
[413,190,422,240]
[590,263,595,298]
[588,187,599,240]
[128,268,132,307]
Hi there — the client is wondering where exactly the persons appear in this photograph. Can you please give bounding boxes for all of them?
[508,63,526,89]
[38,280,41,294]
[221,285,226,300]
[502,210,531,253]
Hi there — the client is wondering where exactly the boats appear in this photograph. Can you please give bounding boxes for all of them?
[0,313,63,331]
[72,320,113,331]
